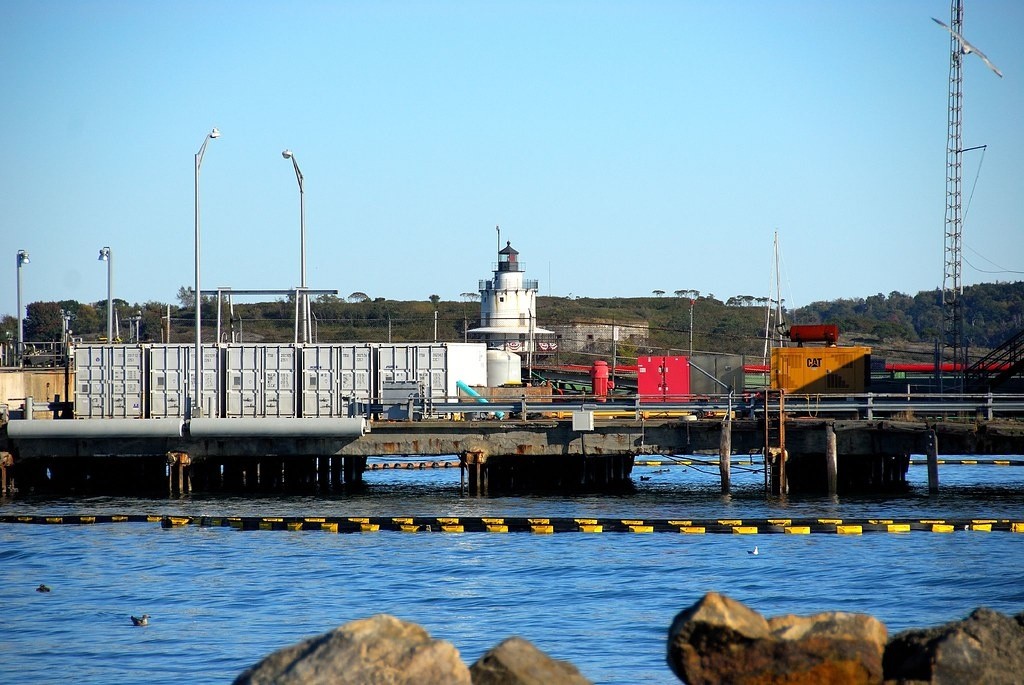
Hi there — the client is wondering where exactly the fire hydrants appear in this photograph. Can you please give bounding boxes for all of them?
[591,360,615,403]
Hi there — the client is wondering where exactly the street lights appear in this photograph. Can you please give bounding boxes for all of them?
[281,149,307,342]
[17,249,32,367]
[98,246,113,345]
[194,127,220,418]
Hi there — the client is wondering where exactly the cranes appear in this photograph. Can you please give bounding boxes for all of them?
[933,1,1024,420]
